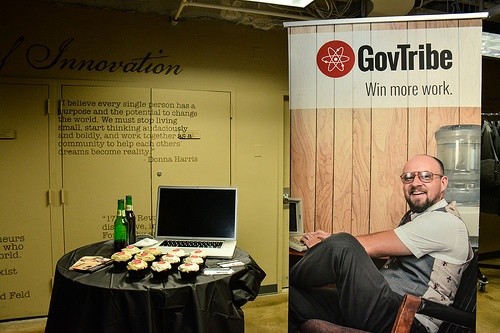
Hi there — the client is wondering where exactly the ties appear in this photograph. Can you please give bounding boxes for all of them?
[399,214,411,227]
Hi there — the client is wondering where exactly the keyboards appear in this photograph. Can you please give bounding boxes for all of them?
[289,235,308,252]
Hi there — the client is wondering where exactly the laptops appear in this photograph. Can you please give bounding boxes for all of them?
[154,186,238,259]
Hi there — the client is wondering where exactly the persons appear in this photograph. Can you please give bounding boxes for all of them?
[288,153,474,333]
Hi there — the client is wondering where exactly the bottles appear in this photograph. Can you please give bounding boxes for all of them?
[114,199,129,251]
[435,124,480,204]
[125,195,136,244]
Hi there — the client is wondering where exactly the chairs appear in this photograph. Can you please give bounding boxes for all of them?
[302,248,476,333]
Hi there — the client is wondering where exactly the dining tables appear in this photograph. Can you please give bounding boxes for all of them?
[45,238,267,333]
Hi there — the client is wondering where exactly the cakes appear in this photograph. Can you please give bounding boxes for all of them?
[111,245,207,283]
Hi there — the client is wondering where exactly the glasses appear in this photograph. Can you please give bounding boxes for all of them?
[400,171,443,184]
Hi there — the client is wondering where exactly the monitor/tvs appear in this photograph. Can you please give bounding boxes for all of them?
[289,202,298,233]
